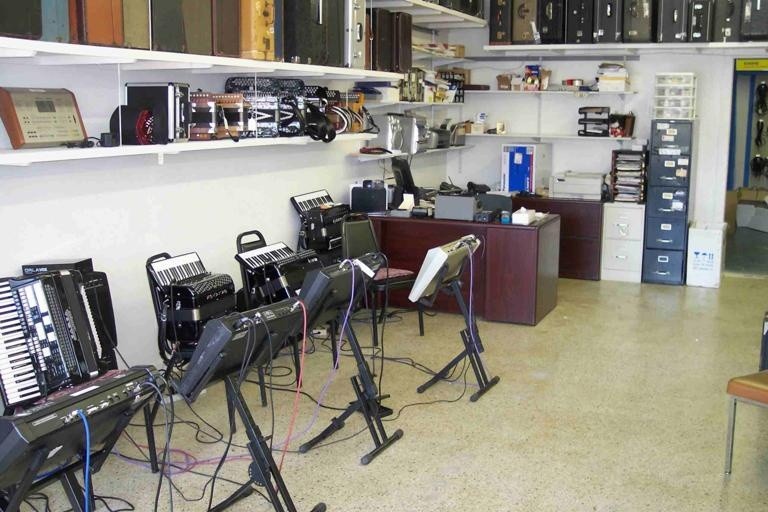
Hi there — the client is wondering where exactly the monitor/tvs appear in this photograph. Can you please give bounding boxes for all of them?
[389,156,420,209]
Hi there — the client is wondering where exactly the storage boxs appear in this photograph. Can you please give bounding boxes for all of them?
[724,186,768,235]
[687,222,727,288]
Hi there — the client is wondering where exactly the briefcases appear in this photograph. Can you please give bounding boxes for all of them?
[0,2,410,72]
[125,82,191,145]
[490,1,767,45]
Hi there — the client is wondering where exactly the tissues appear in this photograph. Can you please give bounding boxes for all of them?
[513,205,537,227]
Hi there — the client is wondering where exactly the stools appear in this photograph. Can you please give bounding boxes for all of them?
[725,370,768,475]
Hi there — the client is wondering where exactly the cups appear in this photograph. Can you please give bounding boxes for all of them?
[499,210,512,225]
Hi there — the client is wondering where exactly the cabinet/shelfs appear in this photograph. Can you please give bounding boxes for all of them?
[653,74,696,120]
[600,203,645,283]
[464,46,636,141]
[514,197,603,281]
[359,0,487,162]
[0,37,405,167]
[610,150,649,203]
[642,120,693,286]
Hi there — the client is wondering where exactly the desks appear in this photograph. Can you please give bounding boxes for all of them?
[360,214,561,327]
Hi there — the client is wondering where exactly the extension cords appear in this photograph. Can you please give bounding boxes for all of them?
[312,328,330,338]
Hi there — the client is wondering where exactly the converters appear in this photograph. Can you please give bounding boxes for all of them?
[101,133,113,147]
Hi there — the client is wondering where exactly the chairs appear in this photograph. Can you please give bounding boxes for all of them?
[237,230,341,387]
[341,214,424,345]
[146,252,267,434]
